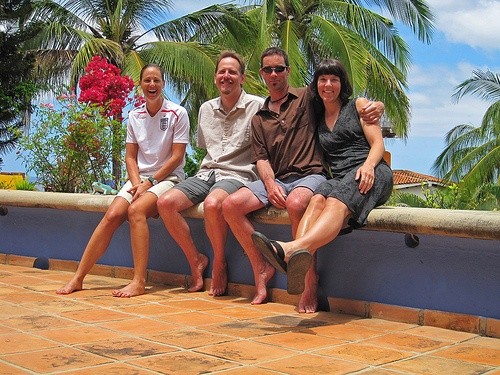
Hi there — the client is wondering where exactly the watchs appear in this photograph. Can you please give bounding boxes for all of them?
[148,176,158,186]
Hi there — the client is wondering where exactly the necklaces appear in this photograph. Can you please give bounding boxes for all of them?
[268,90,289,102]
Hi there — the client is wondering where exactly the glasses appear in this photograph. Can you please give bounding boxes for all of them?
[261,64,289,73]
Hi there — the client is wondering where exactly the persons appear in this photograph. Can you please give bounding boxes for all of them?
[221,46,386,315]
[249,57,394,296]
[157,50,267,297]
[56,63,191,299]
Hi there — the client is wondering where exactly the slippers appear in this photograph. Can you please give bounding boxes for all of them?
[287,249,312,295]
[251,231,287,275]
